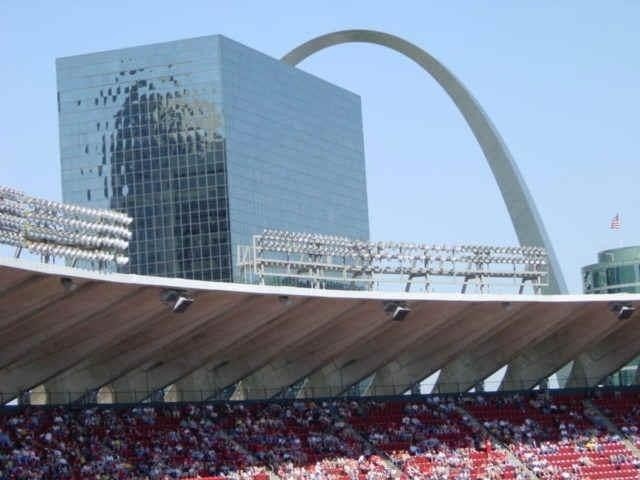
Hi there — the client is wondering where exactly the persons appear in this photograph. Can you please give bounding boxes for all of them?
[0,381,640,480]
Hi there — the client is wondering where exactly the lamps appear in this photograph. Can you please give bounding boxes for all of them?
[163,292,194,316]
[617,306,635,320]
[391,307,410,323]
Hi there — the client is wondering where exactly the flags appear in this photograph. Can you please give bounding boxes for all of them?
[608,216,619,231]
